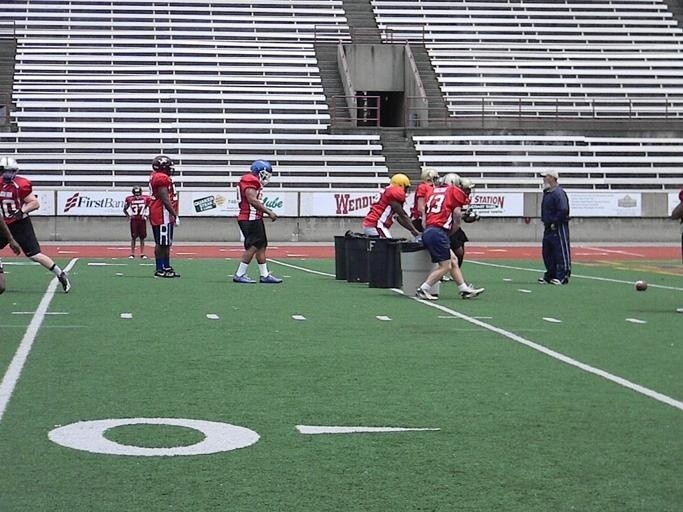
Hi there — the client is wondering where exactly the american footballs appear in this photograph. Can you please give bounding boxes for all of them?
[636,280,647,290]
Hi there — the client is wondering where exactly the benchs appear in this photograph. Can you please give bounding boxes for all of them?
[0,2,682,197]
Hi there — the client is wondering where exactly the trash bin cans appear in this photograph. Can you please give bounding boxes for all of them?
[334,230,440,296]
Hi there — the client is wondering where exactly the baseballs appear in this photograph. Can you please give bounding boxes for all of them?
[469,211,476,218]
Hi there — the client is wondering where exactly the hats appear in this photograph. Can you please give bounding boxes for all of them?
[540,168,558,179]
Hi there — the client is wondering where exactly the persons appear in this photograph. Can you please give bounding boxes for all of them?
[0,156,71,293]
[233,159,283,284]
[0,205,21,295]
[538,170,571,285]
[122,187,150,259]
[362,169,485,300]
[148,155,181,277]
[670,190,683,312]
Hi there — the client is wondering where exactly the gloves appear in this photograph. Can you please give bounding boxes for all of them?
[9,208,24,219]
[460,206,480,222]
[174,215,180,227]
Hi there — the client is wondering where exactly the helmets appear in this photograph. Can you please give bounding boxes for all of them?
[420,168,440,185]
[390,173,411,195]
[151,155,175,177]
[441,173,475,189]
[249,159,273,187]
[0,157,19,181]
[131,185,142,196]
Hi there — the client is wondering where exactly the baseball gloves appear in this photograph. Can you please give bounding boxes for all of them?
[462,206,481,224]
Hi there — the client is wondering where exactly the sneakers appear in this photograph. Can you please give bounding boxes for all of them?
[460,287,485,300]
[57,271,71,293]
[440,275,454,281]
[537,277,568,285]
[260,274,283,283]
[128,254,135,260]
[233,271,256,283]
[140,254,148,259]
[415,287,439,300]
[154,266,180,279]
